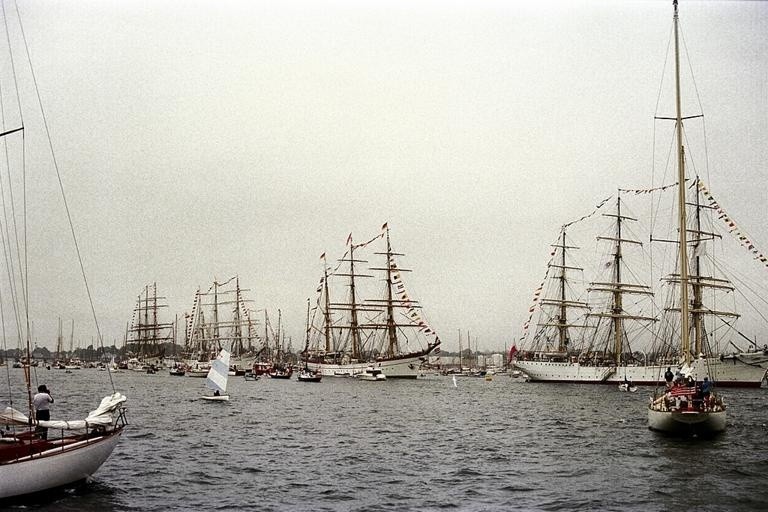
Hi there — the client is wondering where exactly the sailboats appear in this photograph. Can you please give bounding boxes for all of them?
[0,0,128,501]
[199,347,231,403]
[646,9,727,438]
[509,175,768,388]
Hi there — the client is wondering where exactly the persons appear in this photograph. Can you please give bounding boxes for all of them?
[664,366,675,388]
[676,365,683,380]
[720,352,725,362]
[33,383,54,442]
[732,355,738,365]
[702,377,710,400]
